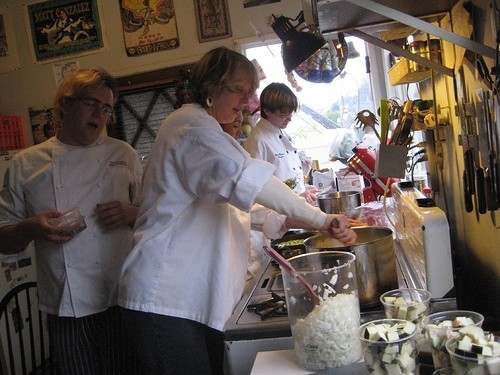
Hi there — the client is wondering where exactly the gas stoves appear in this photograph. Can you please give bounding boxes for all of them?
[220,182,457,341]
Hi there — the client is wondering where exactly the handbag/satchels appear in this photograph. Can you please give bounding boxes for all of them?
[329,120,365,159]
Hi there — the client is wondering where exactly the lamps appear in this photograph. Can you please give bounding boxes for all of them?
[271,11,326,74]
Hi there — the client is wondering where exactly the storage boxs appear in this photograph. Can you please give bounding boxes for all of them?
[0,115,27,153]
[336,173,366,204]
[373,144,408,179]
[386,56,442,86]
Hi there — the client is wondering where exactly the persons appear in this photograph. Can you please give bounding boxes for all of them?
[114,46,359,375]
[0,66,147,375]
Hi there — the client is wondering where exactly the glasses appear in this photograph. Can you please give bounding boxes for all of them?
[273,112,293,119]
[222,83,253,99]
[75,98,113,119]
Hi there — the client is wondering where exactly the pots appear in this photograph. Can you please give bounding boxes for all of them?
[269,231,317,260]
[303,225,399,311]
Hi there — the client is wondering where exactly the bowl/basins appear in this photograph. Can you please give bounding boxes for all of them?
[46,206,87,240]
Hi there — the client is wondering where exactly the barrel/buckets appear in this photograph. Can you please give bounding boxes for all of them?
[316,190,363,215]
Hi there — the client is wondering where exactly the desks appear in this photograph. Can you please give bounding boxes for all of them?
[248,349,370,375]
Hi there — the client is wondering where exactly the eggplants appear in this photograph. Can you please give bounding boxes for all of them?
[363,296,500,375]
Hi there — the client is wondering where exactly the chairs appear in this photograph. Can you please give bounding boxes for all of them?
[0,281,60,375]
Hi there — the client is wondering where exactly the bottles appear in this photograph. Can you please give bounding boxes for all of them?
[402,39,442,73]
[308,159,319,185]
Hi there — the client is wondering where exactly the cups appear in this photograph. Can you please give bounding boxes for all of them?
[421,310,484,369]
[279,250,363,371]
[445,330,500,375]
[356,318,420,375]
[379,287,431,337]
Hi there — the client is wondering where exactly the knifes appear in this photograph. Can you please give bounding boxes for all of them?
[453,84,500,228]
